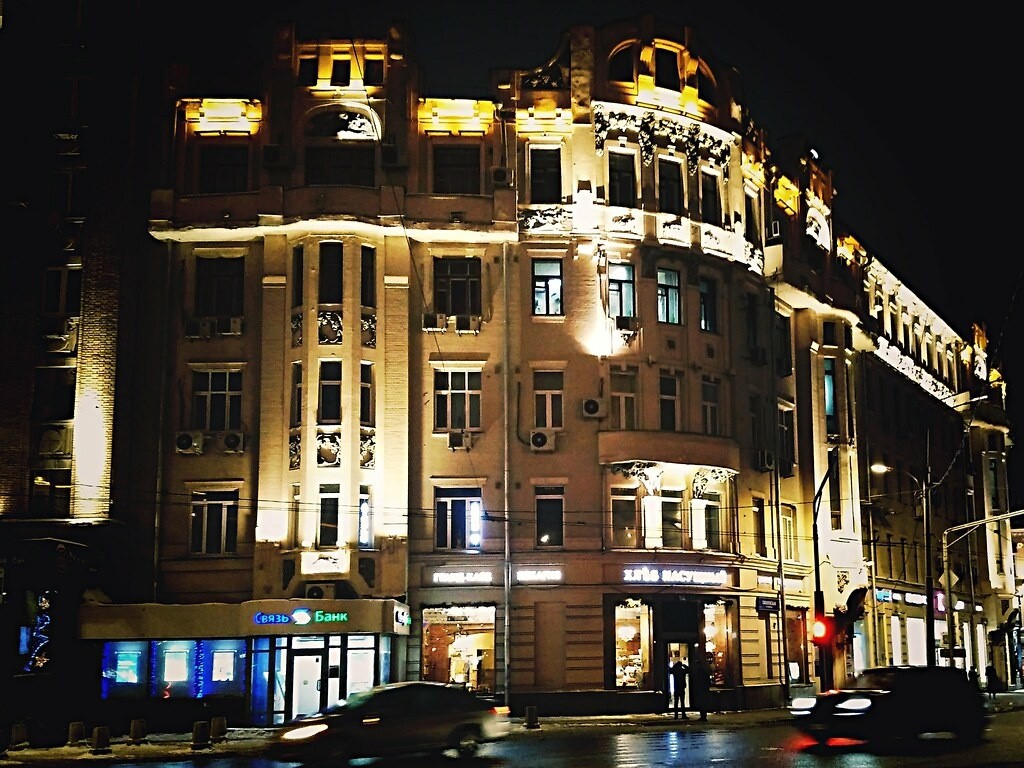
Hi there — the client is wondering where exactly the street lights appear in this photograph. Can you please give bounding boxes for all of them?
[870,460,941,667]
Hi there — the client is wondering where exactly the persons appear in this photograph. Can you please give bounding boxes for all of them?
[681,658,716,722]
[968,660,1024,703]
[668,661,691,721]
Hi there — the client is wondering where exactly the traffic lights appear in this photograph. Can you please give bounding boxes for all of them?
[813,616,831,645]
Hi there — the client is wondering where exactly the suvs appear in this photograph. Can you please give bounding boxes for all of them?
[790,664,990,754]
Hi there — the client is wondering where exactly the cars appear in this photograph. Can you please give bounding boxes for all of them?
[268,675,511,766]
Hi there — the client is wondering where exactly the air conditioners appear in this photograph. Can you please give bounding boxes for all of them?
[529,428,555,452]
[614,316,637,332]
[305,584,337,599]
[447,432,472,450]
[421,313,447,333]
[454,315,480,336]
[174,431,204,455]
[183,317,210,339]
[216,431,244,455]
[758,450,776,471]
[582,398,608,418]
[490,166,514,185]
[214,316,243,336]
